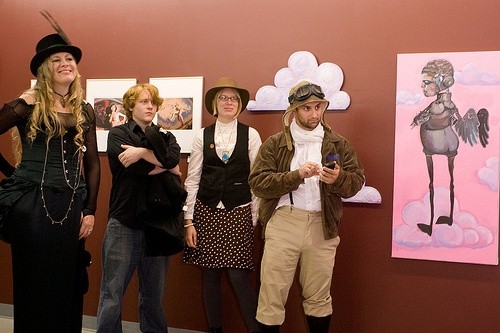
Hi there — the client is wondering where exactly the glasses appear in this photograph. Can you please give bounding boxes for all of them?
[293,83,325,101]
[218,96,240,103]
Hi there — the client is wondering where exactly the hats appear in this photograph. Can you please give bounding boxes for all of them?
[203,77,250,118]
[29,33,82,77]
[281,81,332,151]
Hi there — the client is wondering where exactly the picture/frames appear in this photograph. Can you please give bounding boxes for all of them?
[149,76,203,153]
[390,51,500,266]
[30,80,37,89]
[85,78,137,153]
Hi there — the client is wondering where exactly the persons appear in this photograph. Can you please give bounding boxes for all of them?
[96,84,182,333]
[182,78,264,333]
[0,33,101,333]
[246,80,367,333]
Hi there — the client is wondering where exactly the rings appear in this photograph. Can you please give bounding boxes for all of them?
[308,164,312,169]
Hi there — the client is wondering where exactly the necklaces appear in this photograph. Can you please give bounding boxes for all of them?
[59,127,84,191]
[53,90,71,109]
[40,120,84,226]
[217,118,237,162]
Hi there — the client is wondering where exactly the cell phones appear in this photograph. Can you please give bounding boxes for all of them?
[323,162,335,173]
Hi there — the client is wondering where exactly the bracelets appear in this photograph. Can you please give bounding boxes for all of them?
[184,223,196,228]
